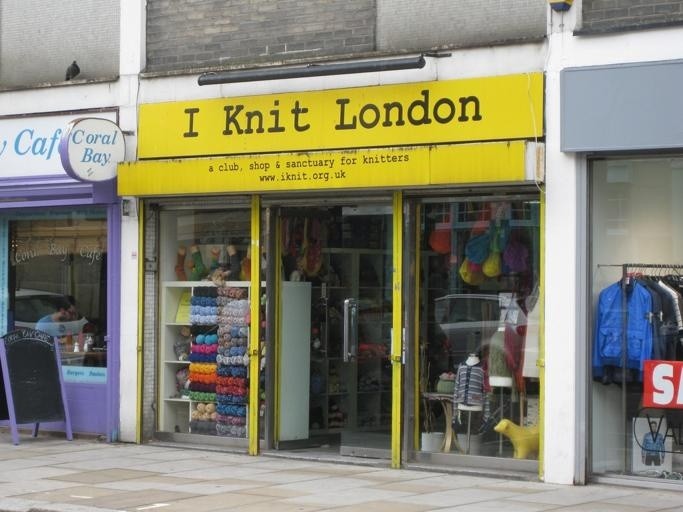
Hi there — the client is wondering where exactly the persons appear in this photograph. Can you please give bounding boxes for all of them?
[457,352,484,412]
[642,422,665,466]
[489,327,512,388]
[35,296,96,344]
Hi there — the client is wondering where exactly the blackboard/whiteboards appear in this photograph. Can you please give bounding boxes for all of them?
[0,327,68,428]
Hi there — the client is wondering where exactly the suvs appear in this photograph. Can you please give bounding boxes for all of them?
[13,286,91,369]
[375,291,528,357]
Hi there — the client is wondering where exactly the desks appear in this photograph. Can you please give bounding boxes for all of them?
[423,393,463,453]
